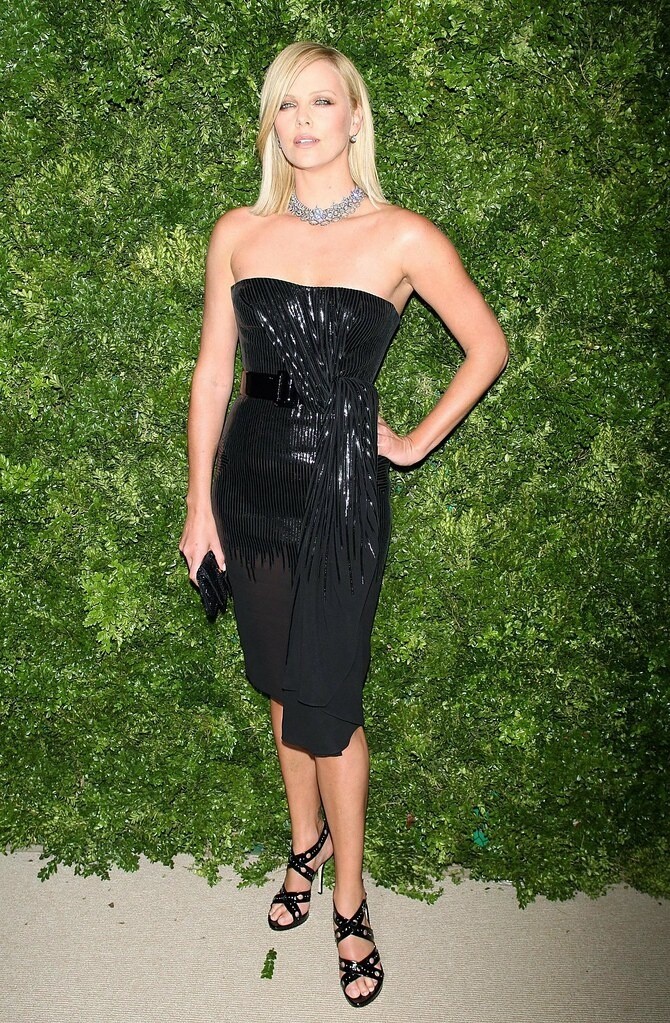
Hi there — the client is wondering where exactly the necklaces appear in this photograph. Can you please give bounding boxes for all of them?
[287,186,368,226]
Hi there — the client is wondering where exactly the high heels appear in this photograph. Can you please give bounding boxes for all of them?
[331,889,384,1007]
[267,819,334,932]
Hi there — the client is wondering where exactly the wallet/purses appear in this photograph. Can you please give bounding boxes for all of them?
[196,550,228,623]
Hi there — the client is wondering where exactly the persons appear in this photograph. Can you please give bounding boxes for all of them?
[176,42,510,1006]
